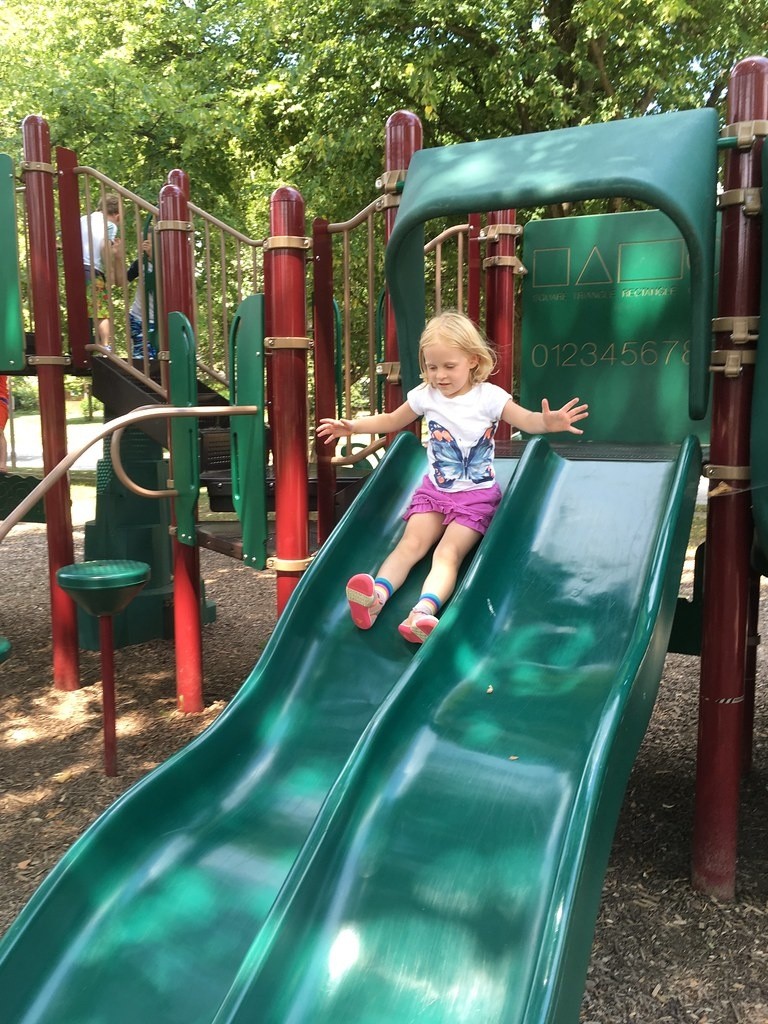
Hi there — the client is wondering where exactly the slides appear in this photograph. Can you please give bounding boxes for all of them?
[0,430,704,1023]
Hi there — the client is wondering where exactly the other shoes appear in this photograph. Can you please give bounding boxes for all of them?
[101,345,116,358]
[398,608,439,644]
[346,573,384,630]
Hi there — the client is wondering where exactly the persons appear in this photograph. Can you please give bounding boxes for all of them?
[109,223,160,361]
[79,195,124,357]
[315,313,589,643]
